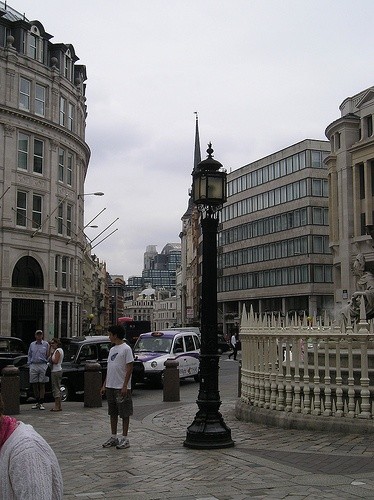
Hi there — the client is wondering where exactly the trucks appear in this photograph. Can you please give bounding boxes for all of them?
[123,320,151,347]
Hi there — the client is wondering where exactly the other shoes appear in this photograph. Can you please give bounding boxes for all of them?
[234,359,238,361]
[228,356,230,359]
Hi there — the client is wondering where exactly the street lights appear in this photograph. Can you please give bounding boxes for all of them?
[84,225,98,229]
[184,139,236,448]
[78,191,105,200]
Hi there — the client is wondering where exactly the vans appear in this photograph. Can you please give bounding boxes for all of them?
[164,327,200,335]
[133,331,201,384]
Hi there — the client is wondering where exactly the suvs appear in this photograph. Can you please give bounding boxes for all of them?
[12,336,137,402]
[0,336,31,403]
[199,333,229,353]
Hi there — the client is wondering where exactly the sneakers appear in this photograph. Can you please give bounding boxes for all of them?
[102,437,130,449]
[31,403,46,410]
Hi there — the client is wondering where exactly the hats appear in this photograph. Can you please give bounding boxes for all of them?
[35,330,43,335]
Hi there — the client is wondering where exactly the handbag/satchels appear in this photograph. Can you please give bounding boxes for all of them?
[45,365,52,378]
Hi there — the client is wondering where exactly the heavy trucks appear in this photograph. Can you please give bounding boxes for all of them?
[118,317,133,325]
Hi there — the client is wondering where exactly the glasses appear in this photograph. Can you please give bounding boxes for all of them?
[50,342,55,345]
[109,333,112,336]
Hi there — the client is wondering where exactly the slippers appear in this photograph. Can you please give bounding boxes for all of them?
[50,408,63,411]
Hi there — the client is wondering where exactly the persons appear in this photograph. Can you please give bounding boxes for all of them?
[0,394,64,500]
[151,340,161,350]
[82,316,305,363]
[100,326,134,449]
[28,330,48,410]
[334,253,374,324]
[46,338,64,411]
